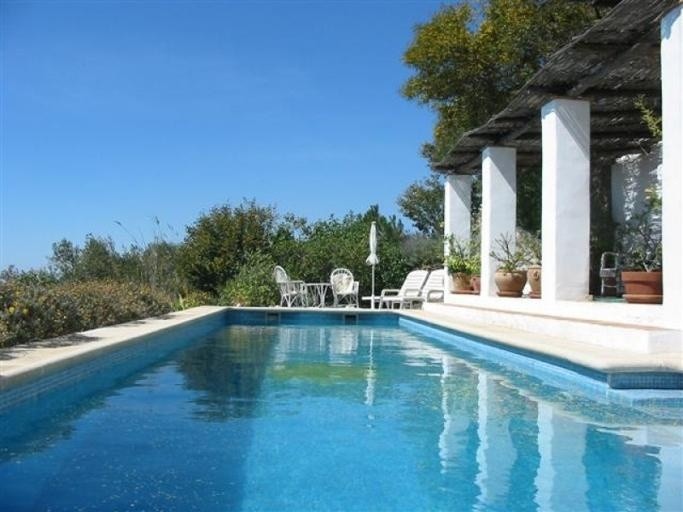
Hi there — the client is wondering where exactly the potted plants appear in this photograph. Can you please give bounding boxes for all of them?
[438,227,541,298]
[618,188,661,303]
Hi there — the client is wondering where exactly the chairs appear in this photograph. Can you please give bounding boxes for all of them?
[269,265,443,312]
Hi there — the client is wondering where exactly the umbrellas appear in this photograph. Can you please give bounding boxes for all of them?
[364,219,380,308]
